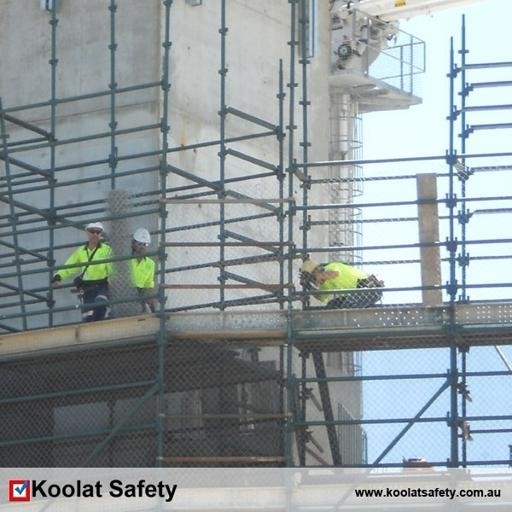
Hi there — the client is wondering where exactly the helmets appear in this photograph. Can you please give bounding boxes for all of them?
[301,259,320,273]
[133,227,151,244]
[85,222,104,231]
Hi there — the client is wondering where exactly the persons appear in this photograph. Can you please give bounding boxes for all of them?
[129,227,155,312]
[298,260,383,310]
[49,221,113,322]
